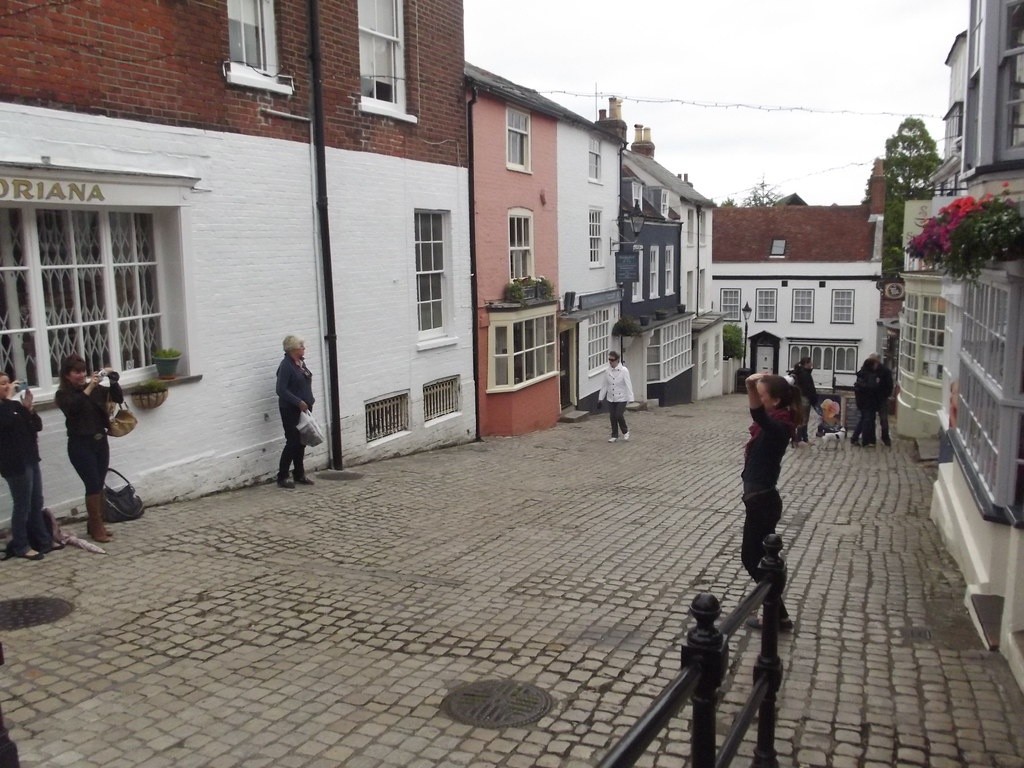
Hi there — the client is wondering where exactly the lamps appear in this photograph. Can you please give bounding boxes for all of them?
[609,200,645,255]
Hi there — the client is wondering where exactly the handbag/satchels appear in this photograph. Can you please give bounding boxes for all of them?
[102,468,145,522]
[107,390,137,437]
[296,409,324,447]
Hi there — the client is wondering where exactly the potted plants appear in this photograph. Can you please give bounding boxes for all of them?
[611,313,643,339]
[149,348,182,380]
[130,380,168,410]
[504,274,558,310]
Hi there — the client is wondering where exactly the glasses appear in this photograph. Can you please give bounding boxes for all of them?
[609,358,617,361]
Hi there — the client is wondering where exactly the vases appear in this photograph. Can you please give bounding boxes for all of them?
[677,304,686,315]
[656,310,668,321]
[639,315,650,327]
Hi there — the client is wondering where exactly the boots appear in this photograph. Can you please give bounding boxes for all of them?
[84,489,114,542]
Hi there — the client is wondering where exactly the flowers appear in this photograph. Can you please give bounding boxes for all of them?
[902,179,1024,288]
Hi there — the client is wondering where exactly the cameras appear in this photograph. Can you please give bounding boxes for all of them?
[17,381,28,390]
[98,370,107,378]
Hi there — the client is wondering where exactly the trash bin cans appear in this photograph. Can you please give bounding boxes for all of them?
[737,368,751,394]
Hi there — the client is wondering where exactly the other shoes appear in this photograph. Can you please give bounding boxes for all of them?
[51,542,63,549]
[25,550,44,560]
[850,438,860,446]
[864,443,876,448]
[296,476,314,484]
[279,479,295,488]
[608,438,618,442]
[880,439,890,448]
[625,432,629,440]
[746,618,793,631]
[799,440,814,446]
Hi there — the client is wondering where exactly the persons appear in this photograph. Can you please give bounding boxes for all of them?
[275,335,315,487]
[740,373,803,630]
[0,371,63,560]
[782,357,822,441]
[851,353,894,446]
[56,355,123,541]
[597,351,634,442]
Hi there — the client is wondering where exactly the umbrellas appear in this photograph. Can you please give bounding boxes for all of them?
[60,533,106,555]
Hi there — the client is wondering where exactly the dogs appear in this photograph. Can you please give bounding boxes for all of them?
[817,426,848,451]
